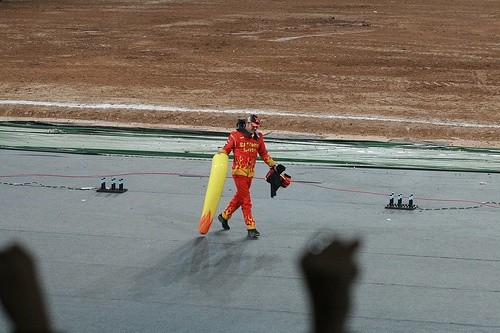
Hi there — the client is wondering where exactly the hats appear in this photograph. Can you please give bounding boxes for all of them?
[245,114,261,127]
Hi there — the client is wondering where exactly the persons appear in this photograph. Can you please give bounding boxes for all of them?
[218,114,277,238]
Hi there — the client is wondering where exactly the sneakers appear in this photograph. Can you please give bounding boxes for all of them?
[218,213,231,231]
[248,229,260,238]
